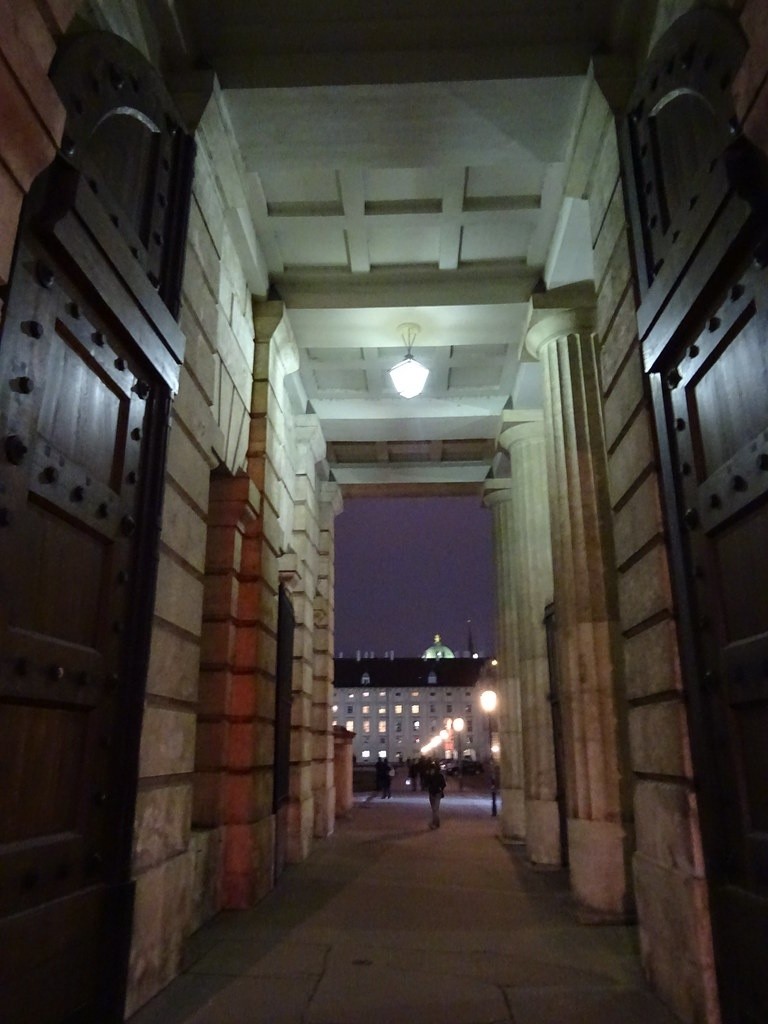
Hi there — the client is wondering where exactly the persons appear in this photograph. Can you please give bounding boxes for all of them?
[381,758,394,799]
[398,753,485,793]
[424,761,447,830]
[373,757,386,793]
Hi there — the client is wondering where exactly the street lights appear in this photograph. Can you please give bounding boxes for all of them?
[453,717,465,792]
[480,688,499,816]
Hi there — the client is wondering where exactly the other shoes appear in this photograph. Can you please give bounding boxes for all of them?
[430,824,438,829]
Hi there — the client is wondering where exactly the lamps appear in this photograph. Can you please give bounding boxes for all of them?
[386,322,431,400]
